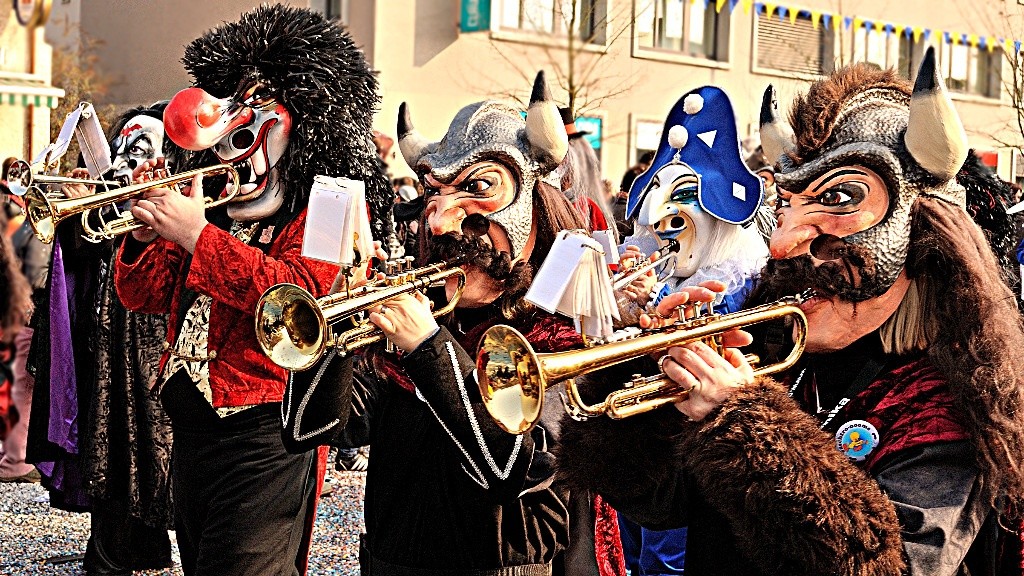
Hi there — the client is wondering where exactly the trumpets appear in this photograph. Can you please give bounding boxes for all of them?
[608,237,683,308]
[6,158,125,202]
[475,287,833,433]
[253,253,469,371]
[23,160,249,247]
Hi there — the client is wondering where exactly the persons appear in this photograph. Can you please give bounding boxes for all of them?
[556,107,658,243]
[559,46,1024,576]
[115,2,398,576]
[1,156,51,484]
[591,86,770,575]
[277,72,591,576]
[23,99,173,576]
[370,130,427,231]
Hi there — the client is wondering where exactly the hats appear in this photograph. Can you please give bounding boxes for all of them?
[625,85,767,227]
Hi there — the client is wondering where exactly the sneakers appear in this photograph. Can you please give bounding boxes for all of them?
[336,453,369,476]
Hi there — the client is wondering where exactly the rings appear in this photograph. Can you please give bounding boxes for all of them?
[657,307,674,320]
[657,354,673,374]
[688,378,699,392]
[379,306,388,318]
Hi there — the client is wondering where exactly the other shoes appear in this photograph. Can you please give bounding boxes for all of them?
[0,466,41,484]
[320,482,334,495]
